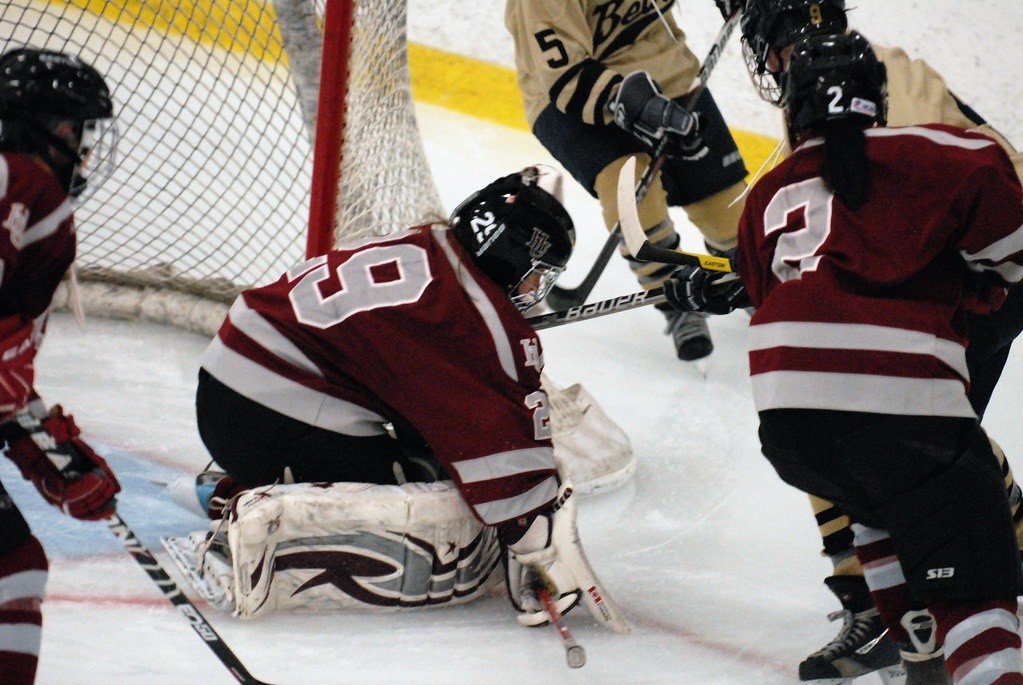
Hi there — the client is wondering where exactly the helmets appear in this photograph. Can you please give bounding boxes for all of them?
[784,30,889,138]
[741,0,848,108]
[449,168,574,314]
[0,48,118,196]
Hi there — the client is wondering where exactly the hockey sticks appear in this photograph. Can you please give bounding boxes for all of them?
[524,262,751,330]
[545,2,744,311]
[531,572,586,670]
[20,403,281,685]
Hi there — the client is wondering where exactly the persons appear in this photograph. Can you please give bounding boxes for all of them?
[1,45,125,685]
[740,0,1021,685]
[159,169,637,632]
[503,0,750,363]
[735,32,1021,685]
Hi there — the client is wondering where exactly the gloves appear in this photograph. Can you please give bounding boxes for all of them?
[613,69,708,163]
[715,0,748,20]
[508,516,580,627]
[3,404,122,521]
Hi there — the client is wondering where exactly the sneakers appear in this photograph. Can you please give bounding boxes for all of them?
[664,307,714,377]
[898,609,951,685]
[798,575,904,685]
